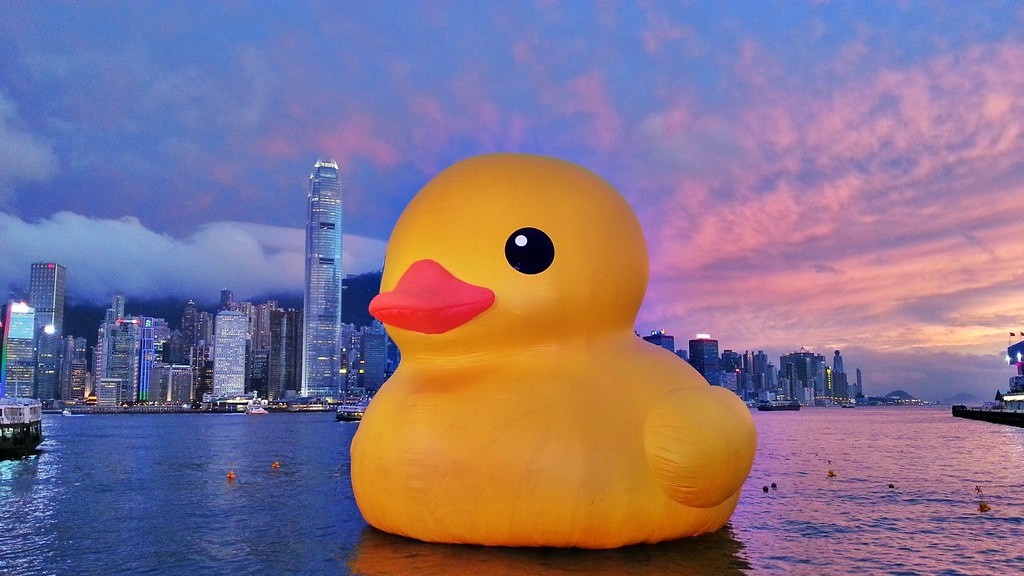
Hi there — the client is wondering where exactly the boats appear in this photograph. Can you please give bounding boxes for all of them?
[335,409,364,422]
[0,402,47,455]
[757,395,802,411]
[288,401,330,411]
[246,405,269,415]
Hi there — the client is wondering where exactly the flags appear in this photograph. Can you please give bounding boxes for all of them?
[1010,333,1015,336]
[1021,332,1024,336]
